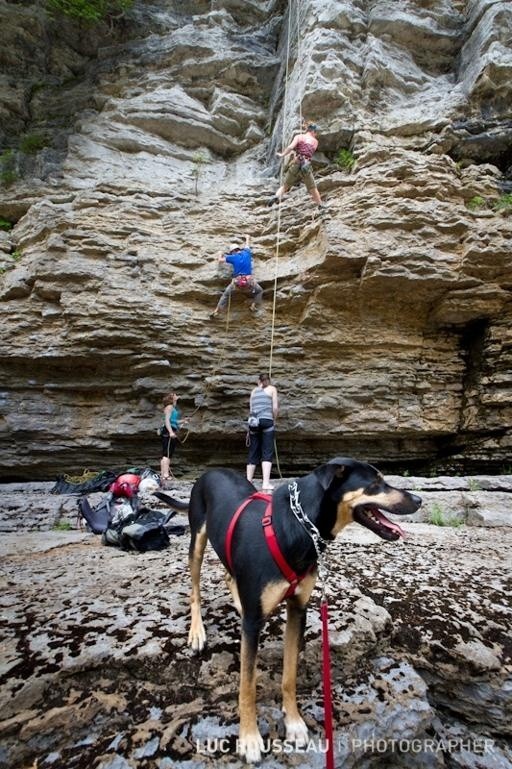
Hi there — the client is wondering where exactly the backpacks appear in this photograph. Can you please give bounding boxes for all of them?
[131,507,185,535]
[110,464,162,498]
[101,516,170,553]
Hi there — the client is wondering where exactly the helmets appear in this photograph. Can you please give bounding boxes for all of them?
[229,243,240,252]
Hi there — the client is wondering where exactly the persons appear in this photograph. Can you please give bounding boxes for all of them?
[158,392,191,481]
[245,374,279,490]
[269,123,323,208]
[207,235,263,317]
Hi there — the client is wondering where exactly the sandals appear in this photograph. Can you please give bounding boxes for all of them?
[161,475,176,481]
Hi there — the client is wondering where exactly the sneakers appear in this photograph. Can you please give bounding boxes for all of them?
[317,204,328,210]
[268,195,278,208]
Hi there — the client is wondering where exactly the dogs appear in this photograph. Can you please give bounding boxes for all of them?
[186,456,423,765]
